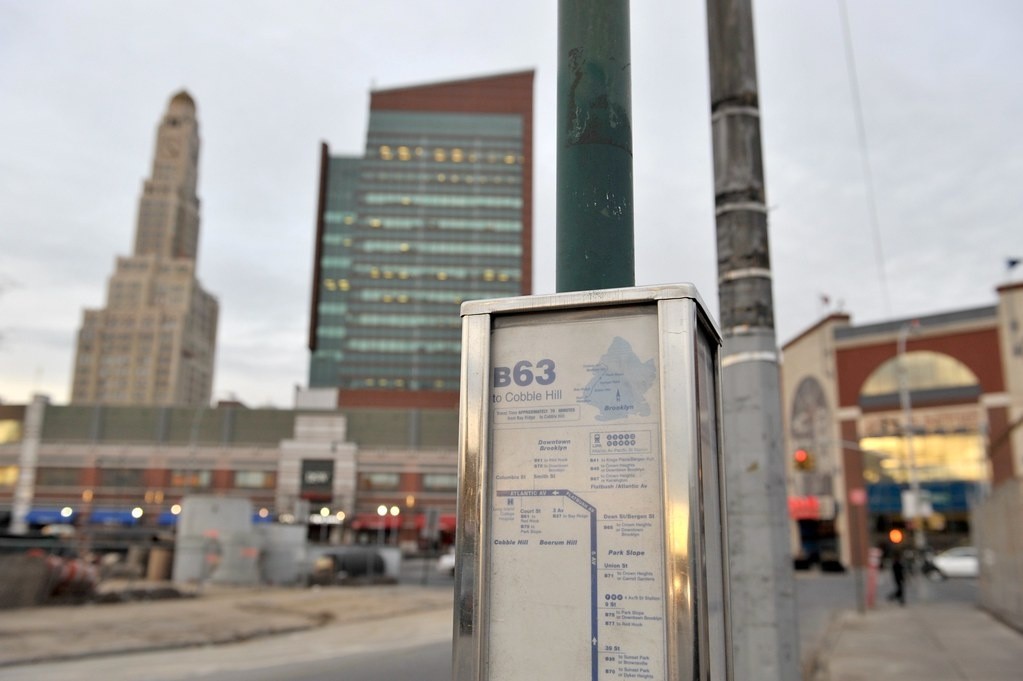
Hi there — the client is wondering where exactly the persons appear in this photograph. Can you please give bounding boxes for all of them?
[889,556,908,605]
